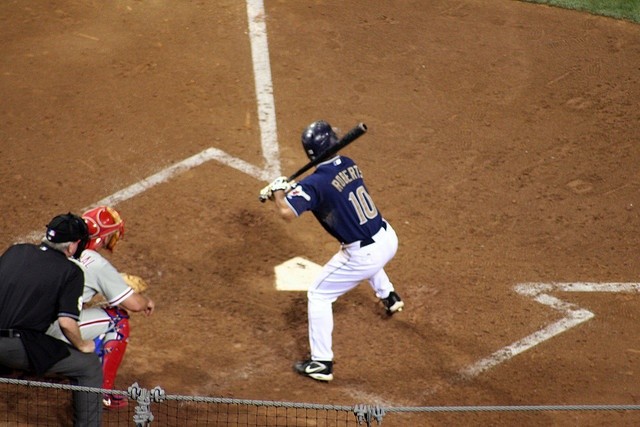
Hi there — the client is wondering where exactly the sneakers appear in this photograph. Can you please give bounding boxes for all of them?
[101,393,130,410]
[381,292,404,316]
[294,359,333,381]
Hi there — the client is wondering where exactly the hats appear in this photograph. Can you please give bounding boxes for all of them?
[46,213,89,259]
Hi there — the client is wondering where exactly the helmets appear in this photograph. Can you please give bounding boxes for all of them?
[81,205,125,254]
[302,121,338,160]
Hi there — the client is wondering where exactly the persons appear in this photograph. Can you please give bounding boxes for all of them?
[260,121,404,381]
[45,205,153,408]
[0,212,103,425]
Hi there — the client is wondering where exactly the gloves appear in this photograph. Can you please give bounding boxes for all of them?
[259,177,295,203]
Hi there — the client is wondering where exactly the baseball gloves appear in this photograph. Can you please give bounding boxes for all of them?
[119,271,148,295]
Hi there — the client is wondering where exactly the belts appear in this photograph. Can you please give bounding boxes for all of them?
[361,222,386,248]
[0,330,21,338]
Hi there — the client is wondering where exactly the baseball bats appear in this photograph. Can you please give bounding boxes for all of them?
[259,123,367,202]
